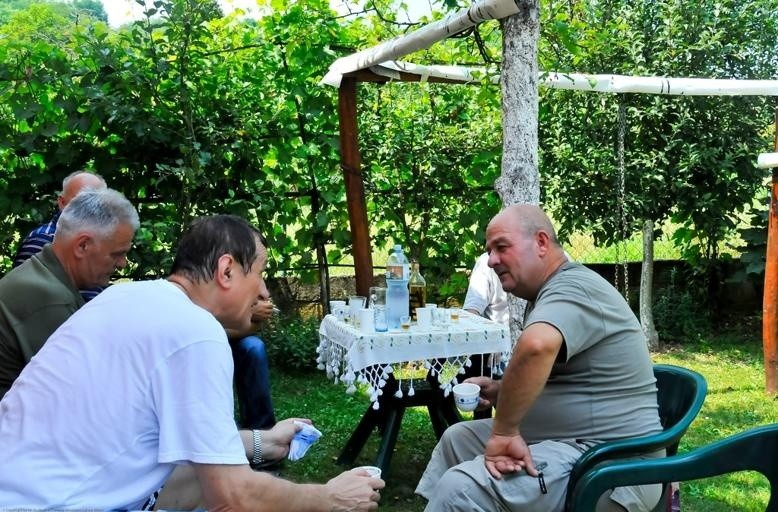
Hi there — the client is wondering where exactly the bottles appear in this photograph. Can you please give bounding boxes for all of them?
[386,245,409,327]
[410,262,427,321]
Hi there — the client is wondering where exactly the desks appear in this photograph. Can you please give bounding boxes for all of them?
[327,306,515,476]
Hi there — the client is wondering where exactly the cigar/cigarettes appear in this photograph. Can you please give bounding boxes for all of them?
[270,307,282,313]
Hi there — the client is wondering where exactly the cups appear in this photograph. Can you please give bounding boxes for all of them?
[355,466,381,490]
[453,384,482,412]
[400,297,459,332]
[330,287,387,332]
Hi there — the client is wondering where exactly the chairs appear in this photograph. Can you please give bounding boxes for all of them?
[562,363,778,512]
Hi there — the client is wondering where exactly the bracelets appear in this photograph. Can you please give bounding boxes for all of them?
[250,429,264,464]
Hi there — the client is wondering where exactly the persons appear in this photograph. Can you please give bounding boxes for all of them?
[1,213,387,512]
[415,199,671,512]
[0,186,140,396]
[220,297,289,472]
[12,171,114,306]
[457,242,576,384]
